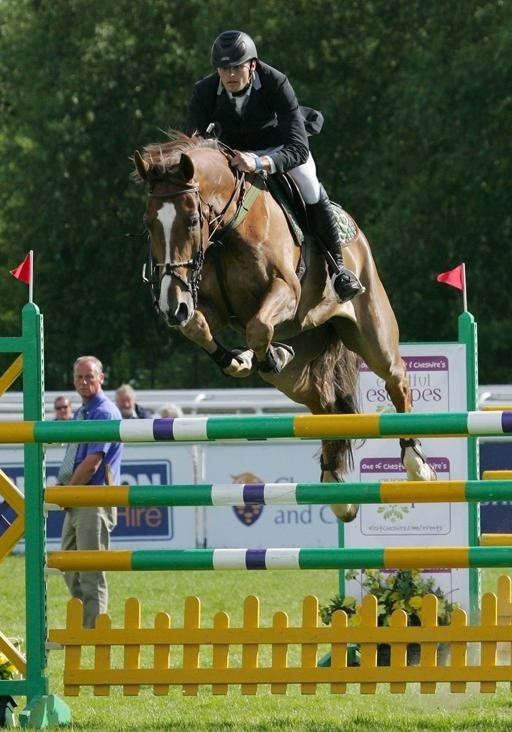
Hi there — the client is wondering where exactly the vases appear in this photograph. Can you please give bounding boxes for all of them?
[0,638,24,681]
[318,569,461,627]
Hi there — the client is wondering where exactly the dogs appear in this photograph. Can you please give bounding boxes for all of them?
[129,128,438,522]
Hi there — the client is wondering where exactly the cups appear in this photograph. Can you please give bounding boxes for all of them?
[55,405,69,410]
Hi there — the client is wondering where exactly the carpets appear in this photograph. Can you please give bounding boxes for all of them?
[55,409,88,487]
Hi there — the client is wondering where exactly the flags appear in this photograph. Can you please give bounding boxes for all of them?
[375,651,451,668]
[0,696,17,728]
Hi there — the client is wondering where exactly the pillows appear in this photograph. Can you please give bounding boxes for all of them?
[208,30,259,69]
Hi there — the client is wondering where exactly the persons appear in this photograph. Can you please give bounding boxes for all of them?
[185,29,364,302]
[115,383,152,420]
[56,355,125,628]
[157,403,184,419]
[48,395,77,422]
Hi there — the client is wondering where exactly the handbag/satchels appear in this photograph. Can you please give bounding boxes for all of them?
[253,156,263,175]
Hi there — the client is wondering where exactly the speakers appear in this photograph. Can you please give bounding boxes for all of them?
[303,183,364,304]
